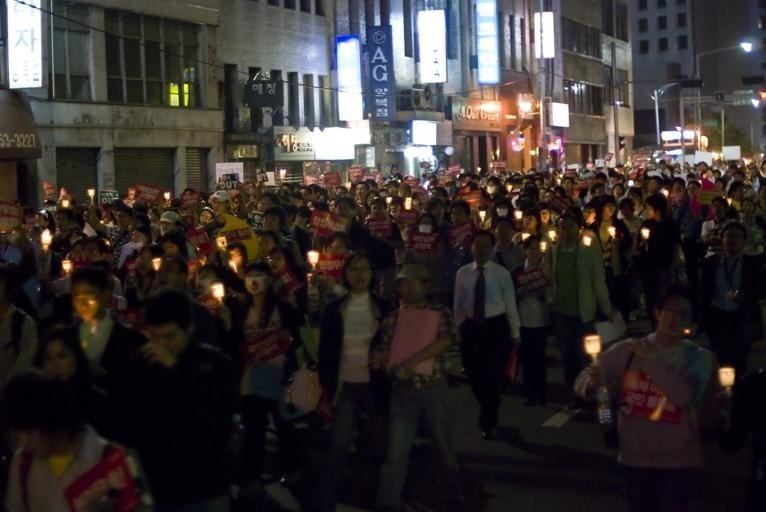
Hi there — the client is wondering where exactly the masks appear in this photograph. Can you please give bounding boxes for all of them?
[244,276,273,294]
[195,278,223,294]
[419,224,432,233]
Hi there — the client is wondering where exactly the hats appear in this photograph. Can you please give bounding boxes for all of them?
[160,211,179,224]
[201,207,215,215]
[209,190,231,207]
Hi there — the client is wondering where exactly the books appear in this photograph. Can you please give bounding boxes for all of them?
[595,312,627,344]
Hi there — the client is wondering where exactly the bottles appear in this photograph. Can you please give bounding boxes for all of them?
[595,354,618,447]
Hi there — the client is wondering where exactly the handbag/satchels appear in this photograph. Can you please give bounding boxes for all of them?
[597,353,634,449]
[290,363,322,411]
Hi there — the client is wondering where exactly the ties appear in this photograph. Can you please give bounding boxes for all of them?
[473,267,485,325]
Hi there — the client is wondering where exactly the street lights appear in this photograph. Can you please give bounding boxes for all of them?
[698,42,752,151]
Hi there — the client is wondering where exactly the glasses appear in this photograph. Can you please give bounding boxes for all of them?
[73,292,102,303]
[372,206,385,210]
[594,190,604,194]
[117,214,130,219]
[346,266,371,273]
[662,307,692,319]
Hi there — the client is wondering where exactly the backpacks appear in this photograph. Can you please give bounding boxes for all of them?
[285,362,320,414]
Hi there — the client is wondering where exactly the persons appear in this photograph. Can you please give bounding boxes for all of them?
[503,167,577,205]
[590,183,605,196]
[425,197,445,227]
[40,327,87,381]
[368,263,480,490]
[138,289,239,463]
[115,226,151,281]
[209,190,229,215]
[201,206,214,224]
[629,187,645,218]
[379,173,486,205]
[371,198,386,215]
[612,183,624,200]
[537,202,551,229]
[453,230,520,439]
[487,176,503,199]
[316,253,384,483]
[522,209,551,242]
[9,384,152,512]
[256,192,280,212]
[492,197,514,223]
[159,211,181,234]
[367,191,380,207]
[356,181,369,212]
[125,245,166,304]
[691,222,766,344]
[390,196,404,219]
[2,230,37,284]
[542,215,611,366]
[510,235,547,401]
[574,283,714,477]
[589,159,660,190]
[619,197,642,249]
[67,266,153,374]
[417,214,436,233]
[160,229,189,258]
[449,199,471,223]
[277,180,352,205]
[307,199,320,211]
[213,248,302,492]
[336,196,362,238]
[2,301,35,384]
[701,196,729,257]
[728,181,743,210]
[267,247,295,307]
[110,206,132,263]
[23,192,124,270]
[262,206,302,264]
[712,154,766,193]
[324,231,351,254]
[637,194,679,320]
[587,195,632,318]
[329,198,336,212]
[119,212,156,269]
[662,158,712,189]
[753,185,766,215]
[295,205,310,233]
[648,176,663,193]
[738,200,764,254]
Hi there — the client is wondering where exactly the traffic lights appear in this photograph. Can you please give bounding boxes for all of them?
[679,78,704,88]
